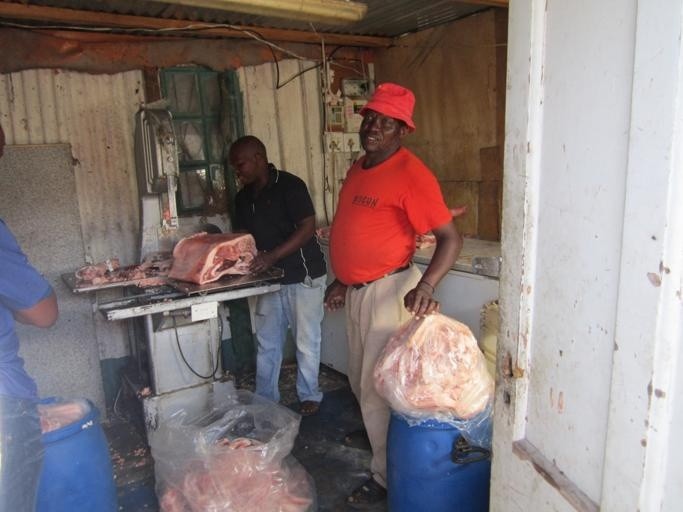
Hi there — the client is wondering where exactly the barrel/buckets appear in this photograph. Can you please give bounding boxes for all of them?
[384,409,492,511]
[34,395,121,512]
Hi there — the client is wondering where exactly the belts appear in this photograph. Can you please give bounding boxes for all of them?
[351,260,414,289]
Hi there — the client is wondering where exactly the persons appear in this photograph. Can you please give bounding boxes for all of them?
[229,136,327,417]
[1,122,59,511]
[323,84,463,508]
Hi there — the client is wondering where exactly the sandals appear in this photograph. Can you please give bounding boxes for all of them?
[298,401,321,418]
[339,430,372,451]
[343,476,387,509]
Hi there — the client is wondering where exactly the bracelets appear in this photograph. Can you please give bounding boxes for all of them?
[418,280,435,294]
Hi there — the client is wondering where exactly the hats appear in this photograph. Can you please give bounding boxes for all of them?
[357,83,416,136]
